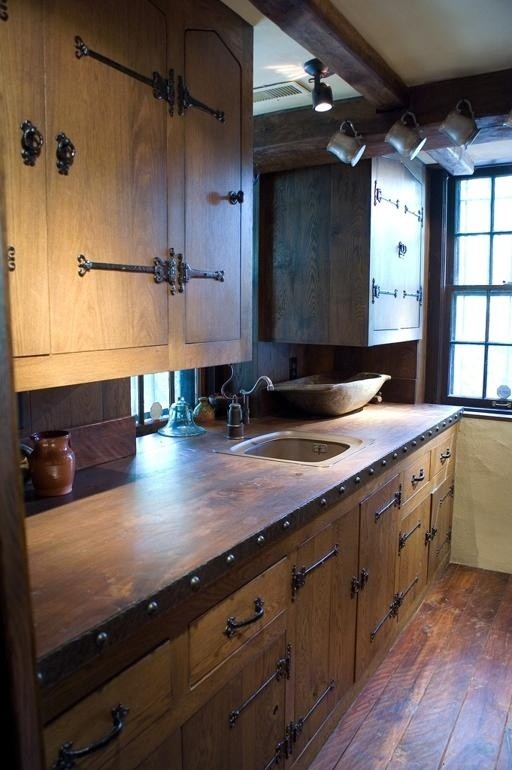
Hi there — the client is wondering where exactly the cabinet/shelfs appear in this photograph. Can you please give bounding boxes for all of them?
[399,424,461,618]
[43,557,291,768]
[294,478,403,753]
[173,1,257,369]
[269,157,425,347]
[0,0,172,368]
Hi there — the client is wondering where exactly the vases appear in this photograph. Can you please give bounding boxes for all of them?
[190,396,215,427]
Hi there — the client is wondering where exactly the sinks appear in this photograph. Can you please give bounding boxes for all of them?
[212,429,375,468]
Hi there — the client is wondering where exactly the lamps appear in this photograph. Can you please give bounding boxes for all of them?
[302,54,337,113]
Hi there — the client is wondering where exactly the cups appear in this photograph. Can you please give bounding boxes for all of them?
[326,99,481,168]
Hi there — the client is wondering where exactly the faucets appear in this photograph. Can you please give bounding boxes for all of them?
[238,376,274,425]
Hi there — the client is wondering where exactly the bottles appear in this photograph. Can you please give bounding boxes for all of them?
[193,397,216,424]
